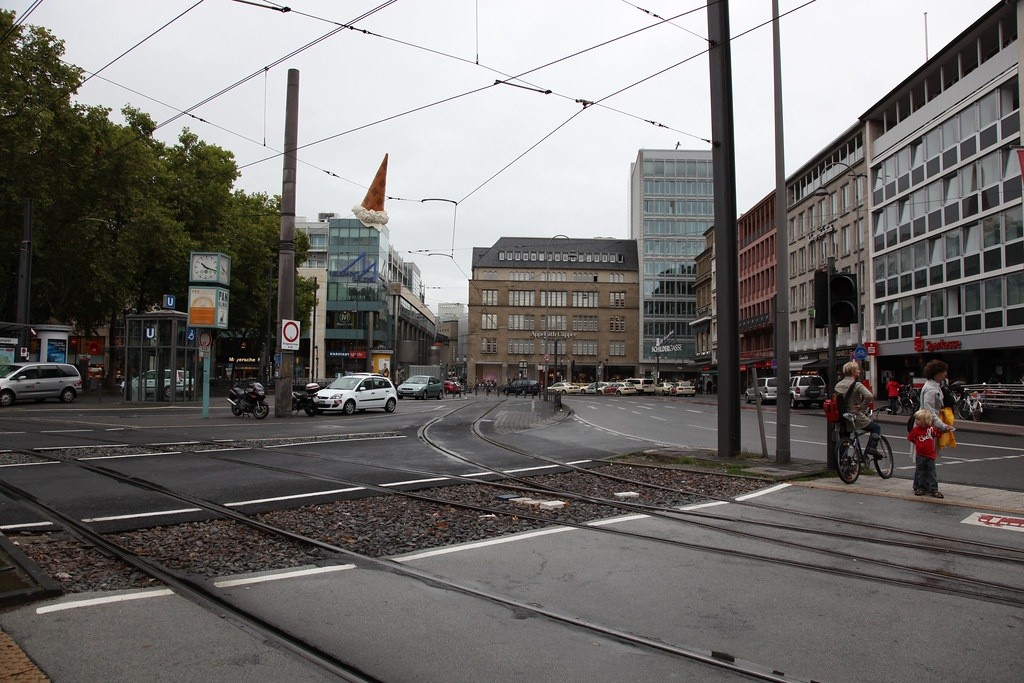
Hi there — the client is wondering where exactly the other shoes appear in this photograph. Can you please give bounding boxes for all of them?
[864,447,885,459]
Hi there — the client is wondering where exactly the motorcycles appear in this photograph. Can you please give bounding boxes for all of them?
[226,382,272,419]
[292,382,320,416]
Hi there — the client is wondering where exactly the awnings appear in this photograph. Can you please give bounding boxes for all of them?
[701,368,718,374]
[788,358,849,371]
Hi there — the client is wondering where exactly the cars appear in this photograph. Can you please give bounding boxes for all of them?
[580,382,609,394]
[316,372,399,413]
[744,376,778,404]
[397,373,444,400]
[655,381,678,396]
[445,381,465,393]
[602,382,637,396]
[675,381,697,396]
[546,380,581,395]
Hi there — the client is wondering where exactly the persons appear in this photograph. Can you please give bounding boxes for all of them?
[706,380,712,395]
[908,359,953,498]
[835,361,883,479]
[885,377,901,415]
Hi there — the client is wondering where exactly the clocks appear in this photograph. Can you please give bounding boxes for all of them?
[190,252,231,287]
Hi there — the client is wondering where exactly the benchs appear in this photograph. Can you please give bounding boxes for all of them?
[962,383,1024,422]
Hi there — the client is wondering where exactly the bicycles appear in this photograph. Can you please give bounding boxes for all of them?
[885,383,922,415]
[834,404,897,484]
[955,389,984,422]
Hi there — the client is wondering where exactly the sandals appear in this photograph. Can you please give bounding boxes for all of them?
[914,489,926,495]
[930,490,944,499]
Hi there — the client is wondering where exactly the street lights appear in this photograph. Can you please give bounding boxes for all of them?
[816,161,866,370]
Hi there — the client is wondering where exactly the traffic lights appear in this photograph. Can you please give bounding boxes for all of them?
[833,272,859,326]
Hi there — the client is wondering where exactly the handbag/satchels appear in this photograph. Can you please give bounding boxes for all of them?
[824,389,847,426]
[907,389,921,433]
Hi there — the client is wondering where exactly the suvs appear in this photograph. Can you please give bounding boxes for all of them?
[502,379,540,397]
[120,367,196,398]
[622,378,655,395]
[790,374,829,408]
[0,361,83,403]
